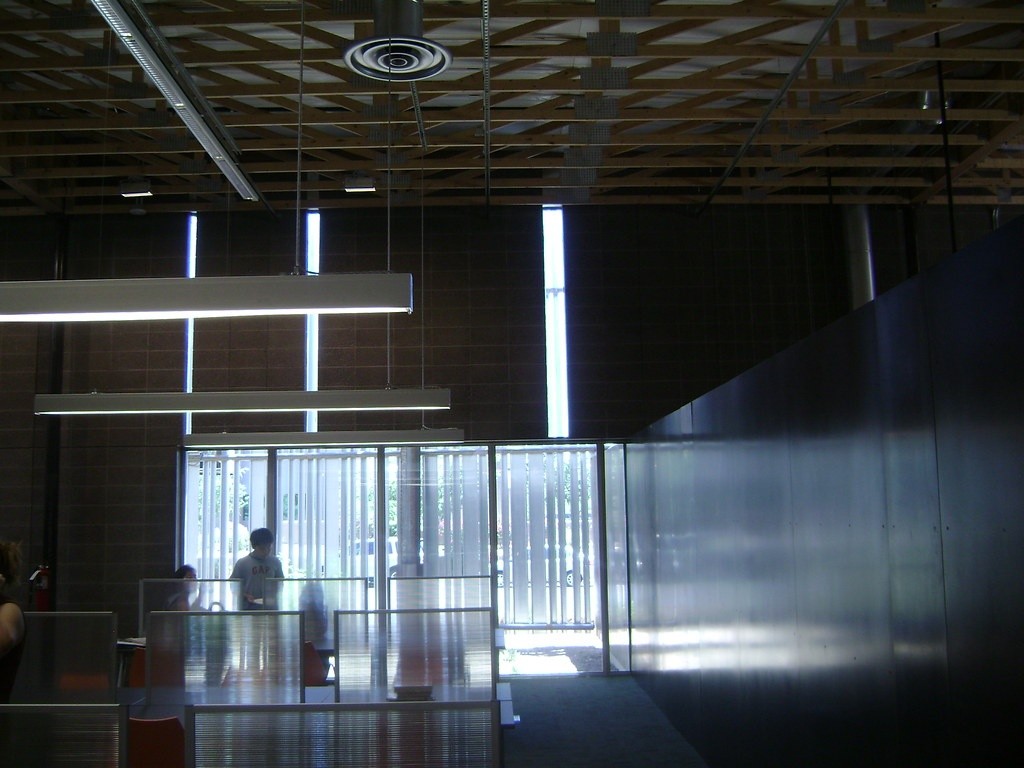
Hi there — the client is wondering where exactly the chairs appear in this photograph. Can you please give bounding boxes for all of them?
[304,641,326,686]
[129,648,178,695]
[128,716,185,768]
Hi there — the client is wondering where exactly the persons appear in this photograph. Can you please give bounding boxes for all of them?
[163,565,210,671]
[0,539,26,707]
[228,528,285,676]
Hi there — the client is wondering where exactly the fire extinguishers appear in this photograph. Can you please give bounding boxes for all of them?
[30,563,50,612]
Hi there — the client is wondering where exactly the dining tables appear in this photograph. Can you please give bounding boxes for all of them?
[116,638,146,689]
[314,628,506,683]
[319,682,515,728]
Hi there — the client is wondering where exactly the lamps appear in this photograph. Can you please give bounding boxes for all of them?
[0,0,413,323]
[184,146,465,450]
[91,0,261,202]
[34,0,452,415]
[305,208,320,433]
[182,210,198,436]
[542,203,569,439]
